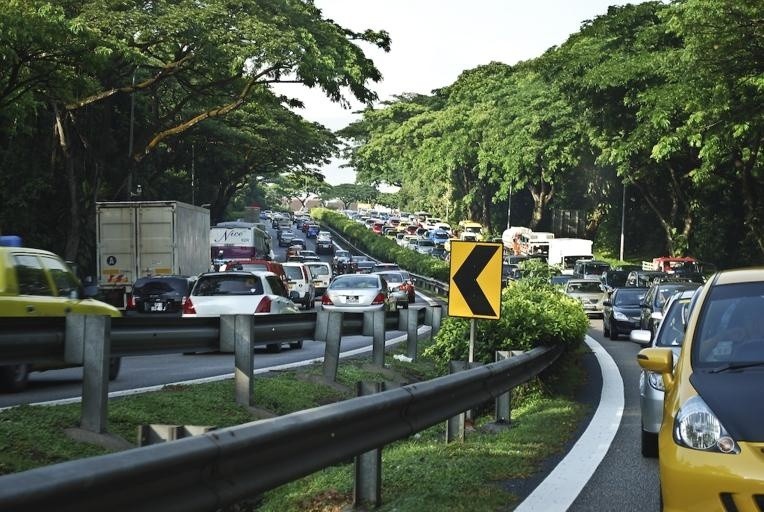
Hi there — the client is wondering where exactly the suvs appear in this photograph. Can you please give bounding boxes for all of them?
[0,235,123,391]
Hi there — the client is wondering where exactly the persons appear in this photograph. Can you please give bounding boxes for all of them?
[699,305,763,365]
